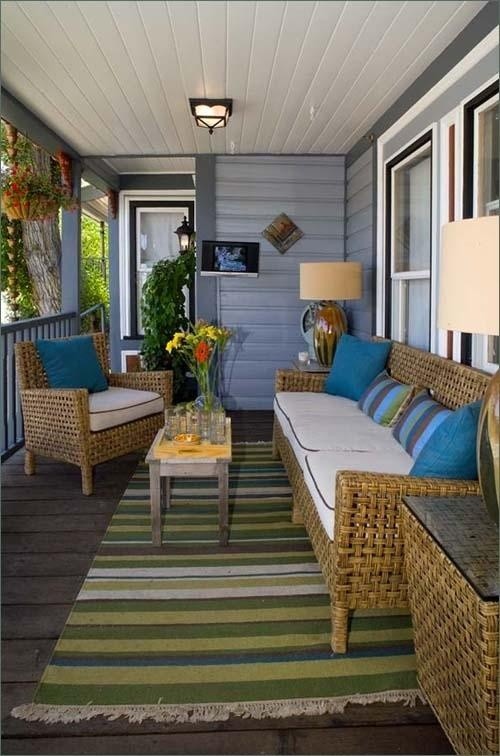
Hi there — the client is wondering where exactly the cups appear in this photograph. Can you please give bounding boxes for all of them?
[299,351,309,364]
[163,404,228,440]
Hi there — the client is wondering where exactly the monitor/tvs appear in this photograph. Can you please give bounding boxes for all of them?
[201,239,261,280]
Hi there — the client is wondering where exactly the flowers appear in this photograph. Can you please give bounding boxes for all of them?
[0,138,79,216]
[165,318,233,413]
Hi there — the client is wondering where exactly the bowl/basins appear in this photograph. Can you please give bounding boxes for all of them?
[172,433,200,447]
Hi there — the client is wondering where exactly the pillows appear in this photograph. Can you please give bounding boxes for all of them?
[31,335,108,394]
[408,398,482,480]
[391,388,455,462]
[357,370,415,428]
[320,330,394,401]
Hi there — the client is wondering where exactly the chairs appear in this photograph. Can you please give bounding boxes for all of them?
[14,332,173,496]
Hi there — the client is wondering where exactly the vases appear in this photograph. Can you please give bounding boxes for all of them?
[0,184,60,223]
[192,392,223,415]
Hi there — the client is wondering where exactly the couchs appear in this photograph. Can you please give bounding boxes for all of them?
[272,335,494,654]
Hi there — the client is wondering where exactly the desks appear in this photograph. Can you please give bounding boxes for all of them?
[399,495,500,755]
[288,358,330,373]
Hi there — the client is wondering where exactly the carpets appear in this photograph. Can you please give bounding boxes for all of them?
[10,440,428,727]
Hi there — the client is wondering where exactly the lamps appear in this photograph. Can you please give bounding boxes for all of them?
[437,214,500,533]
[300,261,363,368]
[174,216,198,401]
[188,97,233,135]
